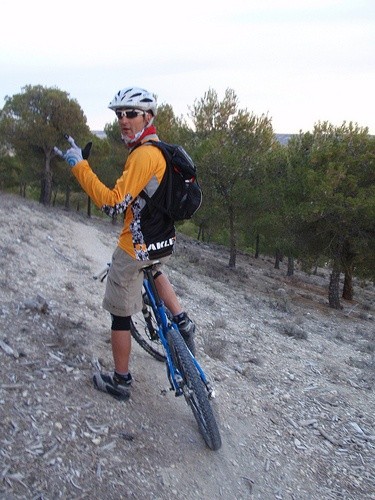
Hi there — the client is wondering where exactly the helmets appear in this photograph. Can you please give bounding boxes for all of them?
[107,87,158,117]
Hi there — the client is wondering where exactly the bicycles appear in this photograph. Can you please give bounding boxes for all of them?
[93,261,225,450]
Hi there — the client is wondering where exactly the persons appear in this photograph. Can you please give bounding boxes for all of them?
[54,86,204,399]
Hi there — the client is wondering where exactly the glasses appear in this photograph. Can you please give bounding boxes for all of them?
[116,109,149,118]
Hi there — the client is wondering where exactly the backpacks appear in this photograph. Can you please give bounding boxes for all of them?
[129,139,204,222]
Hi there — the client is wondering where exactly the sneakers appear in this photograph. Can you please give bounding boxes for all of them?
[174,312,196,339]
[92,373,134,399]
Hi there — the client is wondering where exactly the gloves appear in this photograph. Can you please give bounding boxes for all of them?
[54,133,92,167]
